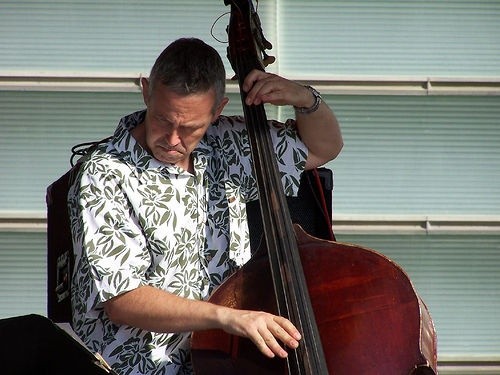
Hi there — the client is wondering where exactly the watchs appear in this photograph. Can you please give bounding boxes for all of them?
[293,83,324,114]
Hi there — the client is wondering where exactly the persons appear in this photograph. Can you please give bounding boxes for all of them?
[64,35,345,374]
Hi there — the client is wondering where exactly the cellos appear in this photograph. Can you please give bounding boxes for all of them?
[189,0,438,375]
